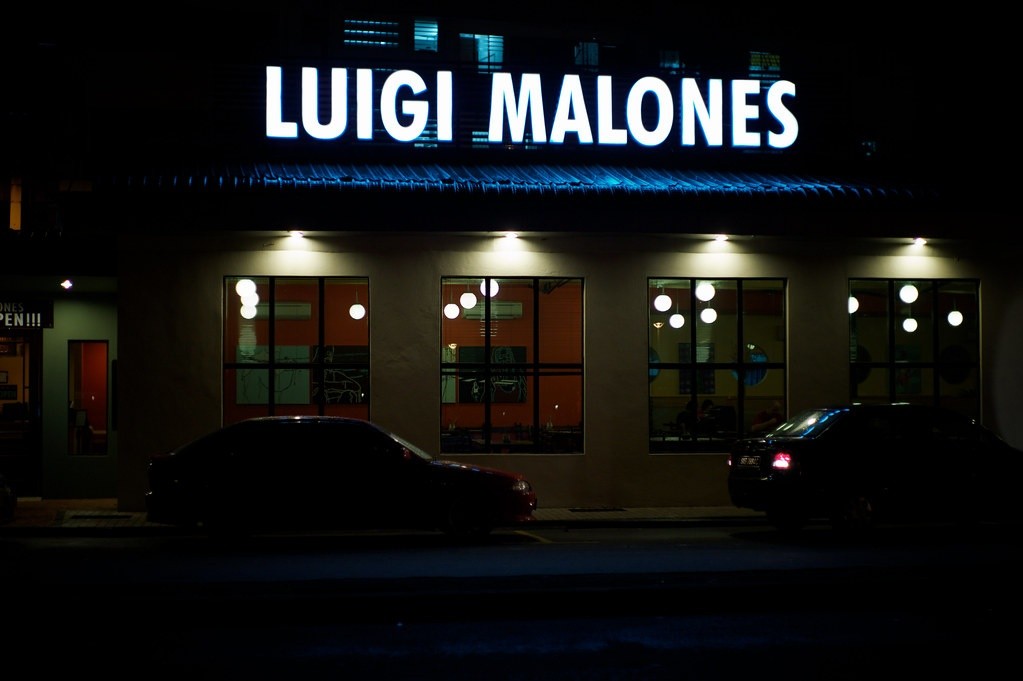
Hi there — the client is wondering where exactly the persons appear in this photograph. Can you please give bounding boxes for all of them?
[676,399,783,435]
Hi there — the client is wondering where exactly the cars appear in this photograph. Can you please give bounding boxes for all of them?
[145,415,538,542]
[726,401,1023,547]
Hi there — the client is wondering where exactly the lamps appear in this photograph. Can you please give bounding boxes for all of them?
[902,304,918,332]
[695,281,716,302]
[947,300,963,326]
[234,277,261,320]
[459,278,478,310]
[669,290,685,329]
[444,278,460,320]
[479,279,499,297]
[899,284,919,304]
[654,287,673,313]
[699,301,718,324]
[348,288,365,320]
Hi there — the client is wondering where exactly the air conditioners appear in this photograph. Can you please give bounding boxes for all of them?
[466,301,523,320]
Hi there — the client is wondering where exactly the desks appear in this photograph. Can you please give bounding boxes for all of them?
[537,430,581,452]
[472,438,532,455]
